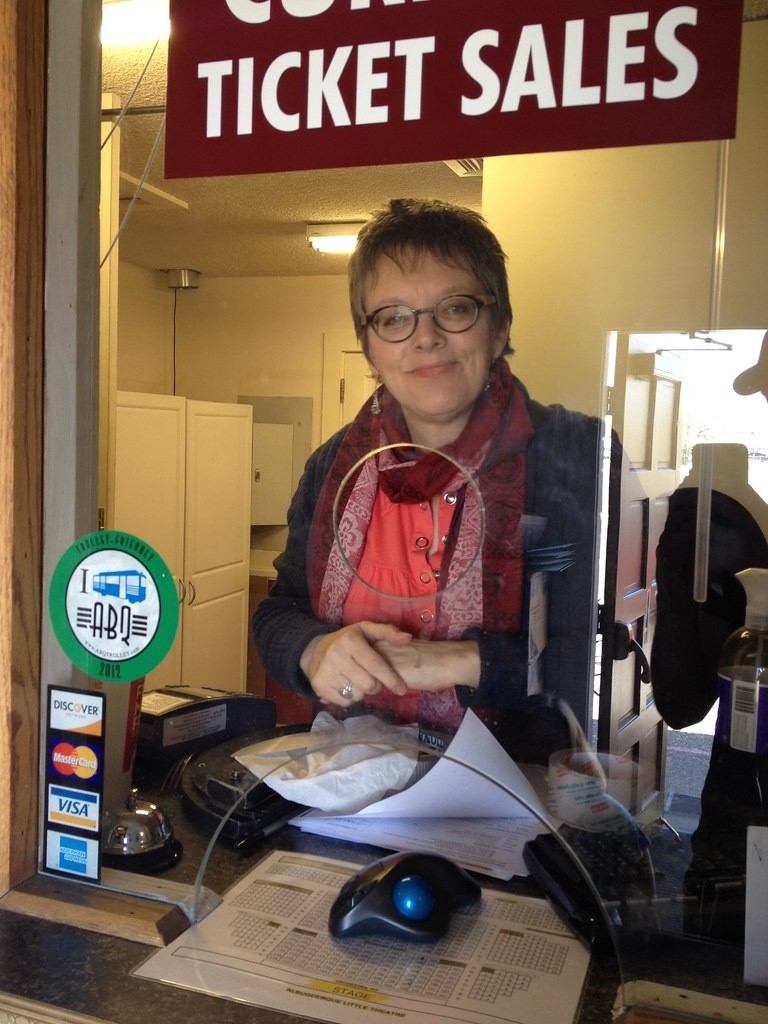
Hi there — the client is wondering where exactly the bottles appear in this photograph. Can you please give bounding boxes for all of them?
[699,565,768,858]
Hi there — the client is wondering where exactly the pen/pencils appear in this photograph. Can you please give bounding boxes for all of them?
[235,810,299,850]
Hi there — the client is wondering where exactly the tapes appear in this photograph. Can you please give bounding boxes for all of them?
[388,729,451,792]
[548,746,656,828]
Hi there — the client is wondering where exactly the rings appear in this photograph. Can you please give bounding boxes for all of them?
[342,683,352,697]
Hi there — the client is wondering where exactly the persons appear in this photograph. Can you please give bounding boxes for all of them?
[252,200,662,762]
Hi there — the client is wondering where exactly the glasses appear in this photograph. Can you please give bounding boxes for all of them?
[361,294,498,343]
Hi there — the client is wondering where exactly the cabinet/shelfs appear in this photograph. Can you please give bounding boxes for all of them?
[106,391,247,698]
